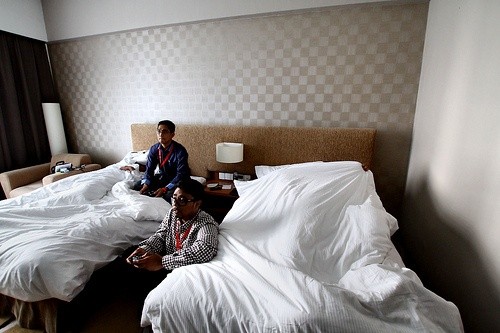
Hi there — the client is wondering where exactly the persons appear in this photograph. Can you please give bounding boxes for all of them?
[131,120,191,205]
[126,178,221,286]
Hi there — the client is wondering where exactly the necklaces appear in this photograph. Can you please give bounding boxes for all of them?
[175,220,192,251]
[158,145,175,167]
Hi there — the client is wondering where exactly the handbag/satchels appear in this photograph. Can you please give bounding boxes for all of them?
[51,161,73,174]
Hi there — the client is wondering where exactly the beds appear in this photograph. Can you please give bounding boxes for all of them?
[0,150,206,333]
[140,161,464,333]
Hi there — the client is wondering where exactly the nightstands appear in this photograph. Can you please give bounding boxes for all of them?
[202,179,238,217]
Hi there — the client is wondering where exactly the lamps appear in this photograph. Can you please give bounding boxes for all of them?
[42,102,67,156]
[216,142,244,163]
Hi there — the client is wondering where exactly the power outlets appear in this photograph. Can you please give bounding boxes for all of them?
[243,175,251,181]
[219,172,224,180]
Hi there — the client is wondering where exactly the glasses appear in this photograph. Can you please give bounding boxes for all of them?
[171,197,197,206]
[157,128,171,134]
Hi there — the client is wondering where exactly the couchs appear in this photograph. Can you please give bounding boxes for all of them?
[0,153,101,198]
